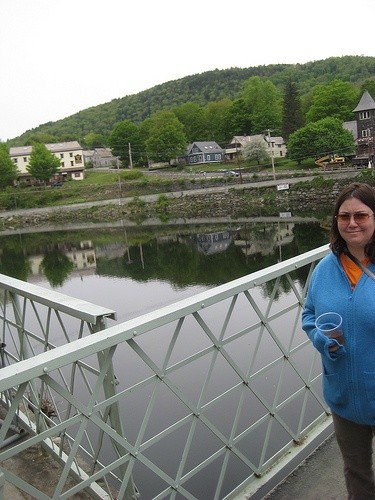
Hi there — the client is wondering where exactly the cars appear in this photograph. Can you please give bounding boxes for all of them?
[51,182,63,186]
[225,170,239,176]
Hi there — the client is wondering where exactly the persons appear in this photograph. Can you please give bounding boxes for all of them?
[301,182,375,500]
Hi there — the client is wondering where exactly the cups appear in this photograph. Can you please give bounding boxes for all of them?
[314,312,344,348]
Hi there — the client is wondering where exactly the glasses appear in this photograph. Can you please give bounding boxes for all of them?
[334,213,374,224]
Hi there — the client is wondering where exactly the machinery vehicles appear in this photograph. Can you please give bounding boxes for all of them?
[314,153,345,169]
